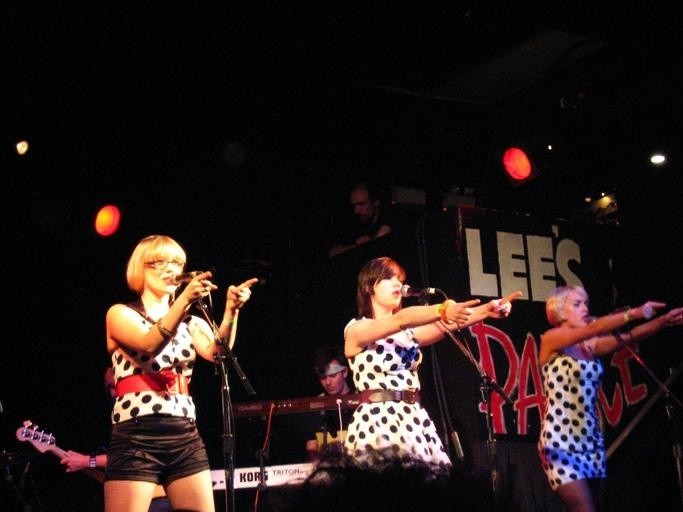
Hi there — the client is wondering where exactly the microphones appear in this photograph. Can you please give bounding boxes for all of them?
[401,285,439,298]
[172,269,204,282]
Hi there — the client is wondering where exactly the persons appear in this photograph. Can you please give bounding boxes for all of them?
[102,235,259,511]
[293,345,356,453]
[325,182,423,259]
[538,284,683,511]
[339,255,521,479]
[59,361,117,475]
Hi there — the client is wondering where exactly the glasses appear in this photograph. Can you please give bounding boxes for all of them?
[144,258,180,270]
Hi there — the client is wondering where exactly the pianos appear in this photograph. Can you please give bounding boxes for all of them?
[230,394,362,419]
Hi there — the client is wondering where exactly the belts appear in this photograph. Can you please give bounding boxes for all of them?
[361,390,421,404]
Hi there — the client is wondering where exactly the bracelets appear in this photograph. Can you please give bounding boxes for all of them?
[623,309,631,322]
[156,317,176,339]
[221,317,237,325]
[436,298,453,325]
